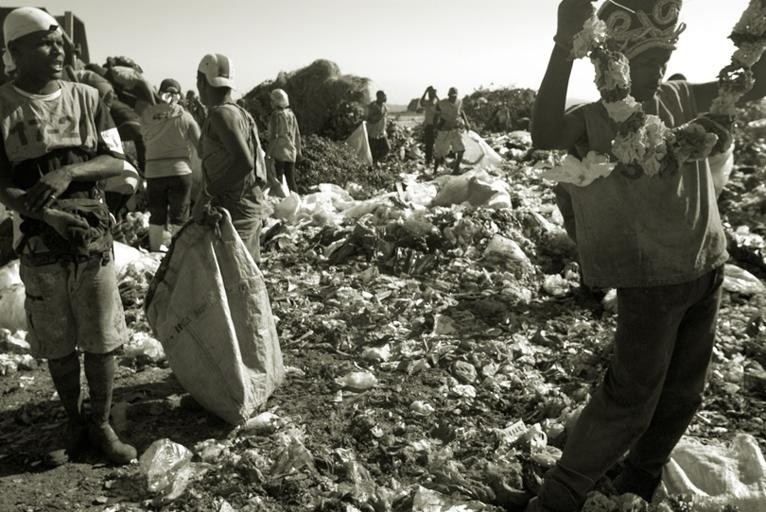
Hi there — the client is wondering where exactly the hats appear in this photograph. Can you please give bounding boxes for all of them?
[3,7,63,50]
[197,53,238,92]
[158,78,181,94]
[595,2,684,63]
[271,89,289,107]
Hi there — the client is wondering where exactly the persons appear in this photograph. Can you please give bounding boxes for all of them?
[0,5,302,468]
[531,1,765,512]
[428,87,471,180]
[420,86,445,167]
[366,89,392,171]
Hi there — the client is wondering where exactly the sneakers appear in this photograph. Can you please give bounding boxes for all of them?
[39,419,88,468]
[90,425,138,464]
